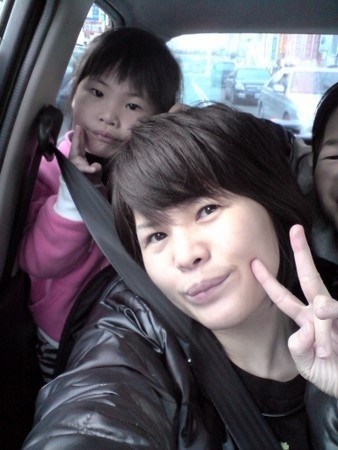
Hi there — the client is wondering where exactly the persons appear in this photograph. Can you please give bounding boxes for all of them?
[18,102,338,449]
[21,19,183,385]
[308,83,338,296]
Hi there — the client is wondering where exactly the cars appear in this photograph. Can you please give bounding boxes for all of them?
[211,60,237,89]
[256,66,338,127]
[224,64,272,105]
